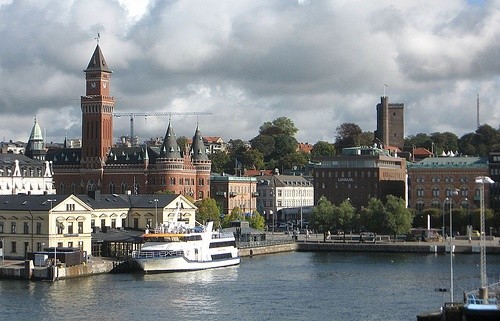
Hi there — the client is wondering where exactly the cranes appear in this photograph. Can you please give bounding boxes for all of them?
[114,112,212,140]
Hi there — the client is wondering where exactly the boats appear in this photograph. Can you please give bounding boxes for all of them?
[133,220,241,274]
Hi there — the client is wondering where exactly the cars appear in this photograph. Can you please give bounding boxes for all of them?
[269,220,311,229]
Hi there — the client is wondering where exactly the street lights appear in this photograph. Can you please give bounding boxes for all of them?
[46,199,56,247]
[442,197,449,237]
[449,189,458,307]
[154,198,159,229]
[474,176,495,302]
[464,197,470,227]
